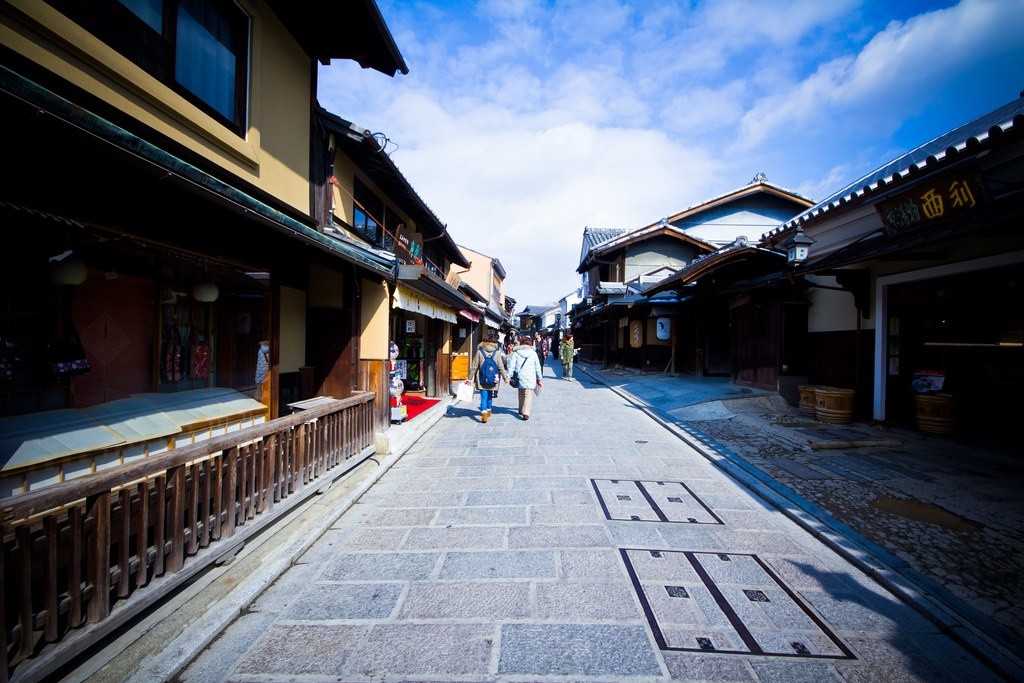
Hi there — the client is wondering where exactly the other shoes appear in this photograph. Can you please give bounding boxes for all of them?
[494,393,497,398]
[519,412,522,415]
[524,415,528,419]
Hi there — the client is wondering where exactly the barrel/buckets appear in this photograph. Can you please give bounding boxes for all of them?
[814,387,857,424]
[797,383,820,415]
[913,393,955,435]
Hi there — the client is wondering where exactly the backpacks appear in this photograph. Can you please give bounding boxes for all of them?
[480,349,498,385]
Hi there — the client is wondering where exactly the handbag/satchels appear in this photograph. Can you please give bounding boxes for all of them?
[457,382,474,402]
[510,372,518,388]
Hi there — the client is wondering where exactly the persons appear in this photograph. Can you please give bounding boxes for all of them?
[534,331,547,377]
[503,333,567,366]
[493,334,505,397]
[255,337,270,403]
[506,337,542,420]
[464,333,510,422]
[560,334,574,382]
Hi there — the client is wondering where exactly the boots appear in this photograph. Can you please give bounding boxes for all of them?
[481,411,488,423]
[487,409,490,417]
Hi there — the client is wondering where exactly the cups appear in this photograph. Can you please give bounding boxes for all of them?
[452,352,454,355]
[460,352,464,355]
[464,352,469,356]
[454,353,457,355]
